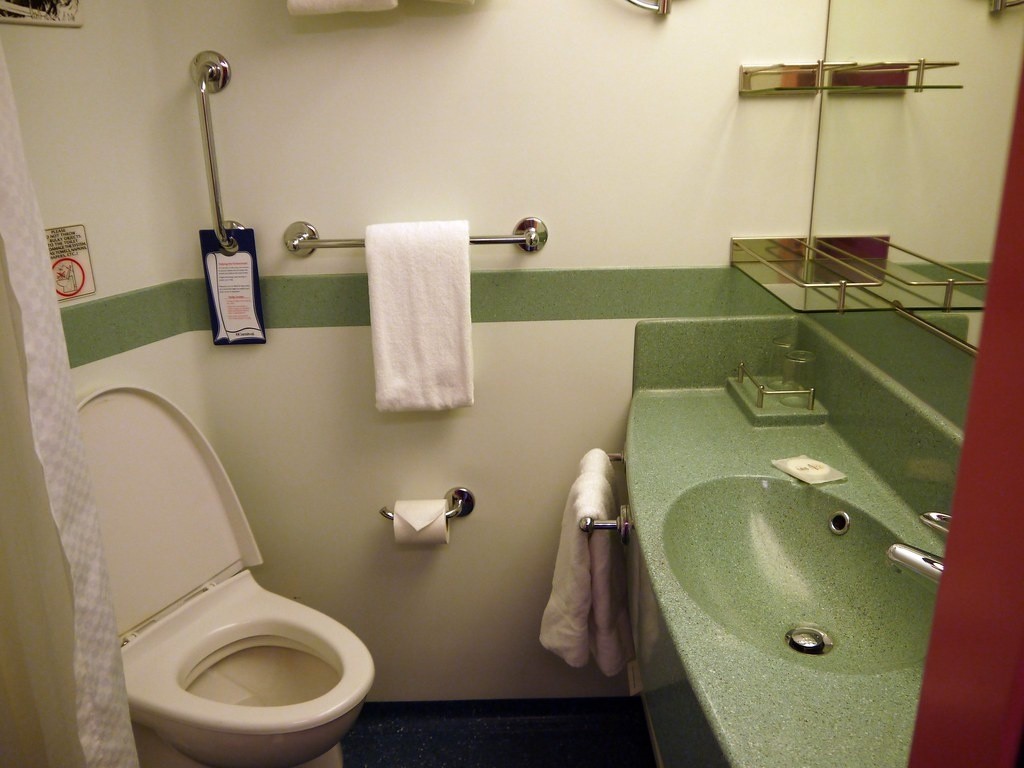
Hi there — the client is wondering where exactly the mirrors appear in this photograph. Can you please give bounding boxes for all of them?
[809,1,1023,350]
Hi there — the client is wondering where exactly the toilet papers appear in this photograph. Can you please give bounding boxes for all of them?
[392,498,451,547]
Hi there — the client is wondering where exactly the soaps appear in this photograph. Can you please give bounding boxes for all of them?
[770,454,849,486]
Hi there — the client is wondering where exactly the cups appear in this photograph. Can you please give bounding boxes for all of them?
[766,336,798,391]
[781,350,817,408]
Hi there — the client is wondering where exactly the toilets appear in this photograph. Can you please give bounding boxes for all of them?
[75,379,379,768]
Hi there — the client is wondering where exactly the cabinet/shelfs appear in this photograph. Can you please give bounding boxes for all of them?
[579,453,730,768]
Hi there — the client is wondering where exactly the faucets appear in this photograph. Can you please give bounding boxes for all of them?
[884,541,945,585]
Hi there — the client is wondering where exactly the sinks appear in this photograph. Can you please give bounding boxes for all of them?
[660,473,941,676]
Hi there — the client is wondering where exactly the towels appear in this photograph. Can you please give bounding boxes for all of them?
[537,447,636,677]
[362,217,479,414]
